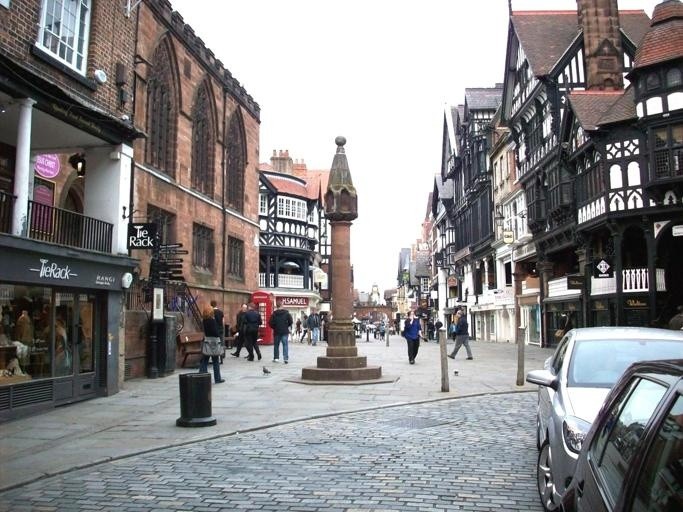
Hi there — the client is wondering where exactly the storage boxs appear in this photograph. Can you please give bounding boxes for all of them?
[177,331,204,368]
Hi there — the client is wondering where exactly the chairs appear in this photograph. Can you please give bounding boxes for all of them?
[410,359,415,364]
[273,358,289,364]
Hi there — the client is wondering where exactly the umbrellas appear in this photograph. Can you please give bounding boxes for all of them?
[448,355,455,359]
[215,379,225,383]
[466,357,473,360]
[231,353,261,361]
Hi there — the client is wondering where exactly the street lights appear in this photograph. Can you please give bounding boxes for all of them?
[202,337,224,355]
[554,329,564,337]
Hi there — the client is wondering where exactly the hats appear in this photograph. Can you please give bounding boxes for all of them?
[526,326,683,512]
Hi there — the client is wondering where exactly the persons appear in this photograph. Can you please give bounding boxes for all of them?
[371,321,387,340]
[402,310,425,364]
[270,300,294,364]
[435,318,443,342]
[449,309,475,360]
[209,300,226,359]
[425,318,436,340]
[195,305,228,383]
[289,307,325,345]
[230,304,247,357]
[14,311,35,365]
[448,321,457,339]
[243,303,263,360]
[43,318,71,374]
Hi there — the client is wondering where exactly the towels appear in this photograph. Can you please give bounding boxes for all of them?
[176,373,216,427]
